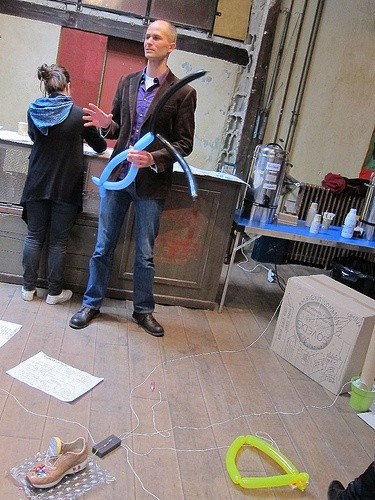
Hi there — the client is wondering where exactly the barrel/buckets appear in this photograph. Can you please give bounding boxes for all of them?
[357,172,375,241]
[240,142,288,224]
[350,375,375,412]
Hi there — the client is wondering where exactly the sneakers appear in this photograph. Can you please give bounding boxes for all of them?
[21,286,35,301]
[46,289,73,305]
[25,436,89,489]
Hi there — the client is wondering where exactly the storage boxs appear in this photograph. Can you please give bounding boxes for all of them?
[271,274,375,394]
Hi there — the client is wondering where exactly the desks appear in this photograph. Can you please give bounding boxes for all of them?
[217,208,375,316]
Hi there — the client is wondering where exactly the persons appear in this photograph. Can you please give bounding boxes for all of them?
[69,19,197,337]
[21,63,108,305]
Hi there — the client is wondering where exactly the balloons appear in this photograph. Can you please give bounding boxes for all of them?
[90,132,155,198]
[226,434,310,492]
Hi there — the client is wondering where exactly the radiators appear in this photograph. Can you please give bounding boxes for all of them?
[287,183,375,271]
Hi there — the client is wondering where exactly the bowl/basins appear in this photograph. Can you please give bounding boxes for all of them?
[353,230,365,238]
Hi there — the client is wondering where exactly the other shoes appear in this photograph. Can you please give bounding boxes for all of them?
[328,480,345,499]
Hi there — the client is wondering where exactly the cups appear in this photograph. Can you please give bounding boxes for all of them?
[18,122,28,136]
[322,216,333,230]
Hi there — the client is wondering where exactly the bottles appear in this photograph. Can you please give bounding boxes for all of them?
[341,208,357,239]
[305,203,318,227]
[309,214,321,234]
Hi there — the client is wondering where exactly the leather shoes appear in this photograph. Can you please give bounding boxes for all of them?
[132,311,164,336]
[69,306,100,328]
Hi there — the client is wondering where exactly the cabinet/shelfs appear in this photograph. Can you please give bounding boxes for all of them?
[0,149,240,310]
